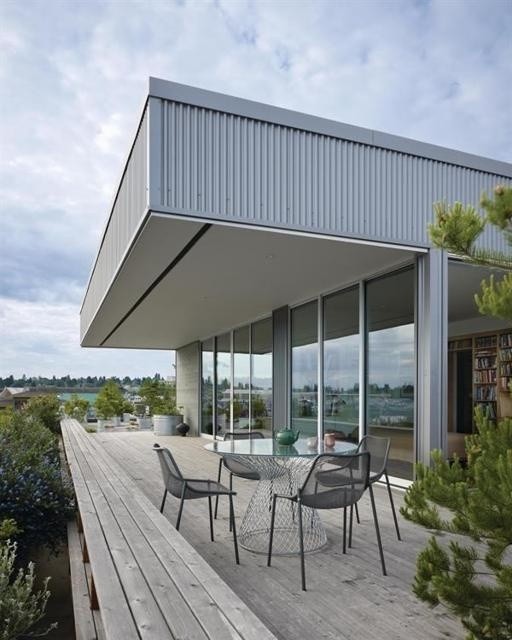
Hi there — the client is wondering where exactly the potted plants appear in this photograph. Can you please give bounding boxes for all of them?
[135,401,152,429]
[94,406,106,433]
[96,376,121,427]
[150,397,184,436]
[122,398,135,423]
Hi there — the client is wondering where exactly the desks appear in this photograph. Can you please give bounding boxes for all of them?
[204,438,361,556]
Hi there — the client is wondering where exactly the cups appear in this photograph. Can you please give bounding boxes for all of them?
[324,433,335,448]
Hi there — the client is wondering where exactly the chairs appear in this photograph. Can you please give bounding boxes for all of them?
[210,433,295,531]
[310,435,401,542]
[267,451,386,590]
[153,442,240,565]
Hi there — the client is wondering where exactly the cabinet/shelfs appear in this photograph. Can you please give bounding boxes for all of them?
[471,328,512,433]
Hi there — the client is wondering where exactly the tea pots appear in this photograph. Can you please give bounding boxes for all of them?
[275,427,300,445]
